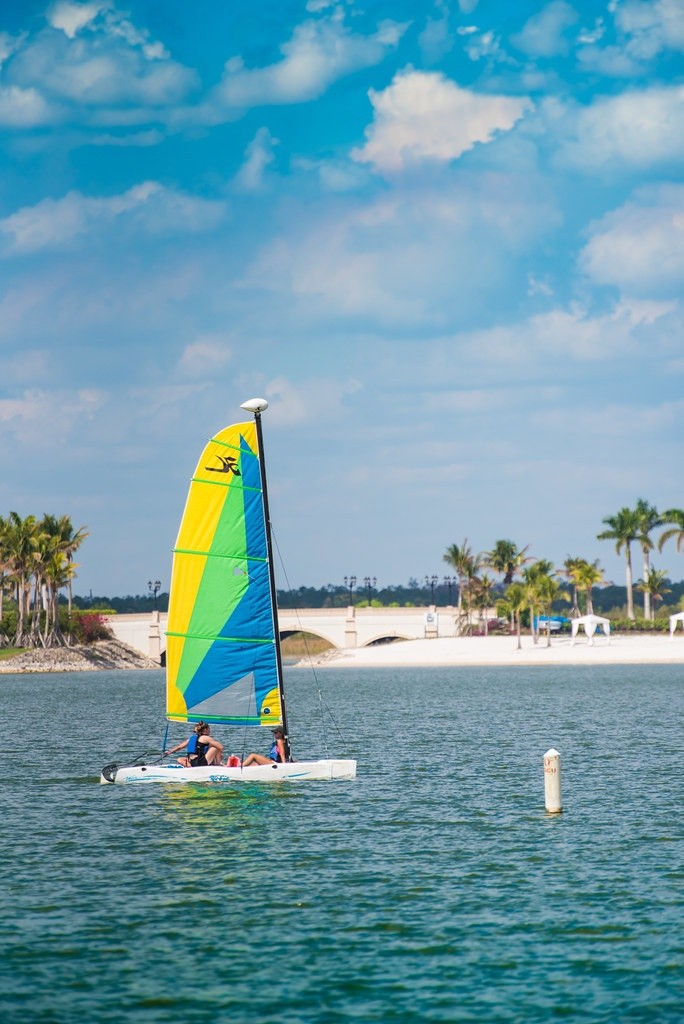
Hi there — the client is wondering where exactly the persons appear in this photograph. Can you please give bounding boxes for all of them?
[241,726,286,766]
[164,721,225,767]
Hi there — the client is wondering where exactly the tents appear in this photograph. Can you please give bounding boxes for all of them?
[570,614,613,645]
[669,611,684,638]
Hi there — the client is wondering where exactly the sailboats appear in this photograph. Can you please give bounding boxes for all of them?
[99,395,357,787]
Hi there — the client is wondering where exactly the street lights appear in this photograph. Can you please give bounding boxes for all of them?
[343,576,357,618]
[363,576,377,611]
[148,579,161,627]
[425,575,439,613]
[443,576,457,613]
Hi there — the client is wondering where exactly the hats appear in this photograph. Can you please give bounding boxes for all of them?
[270,727,284,734]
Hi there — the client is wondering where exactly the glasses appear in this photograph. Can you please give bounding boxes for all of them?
[274,732,278,734]
[193,731,196,733]
